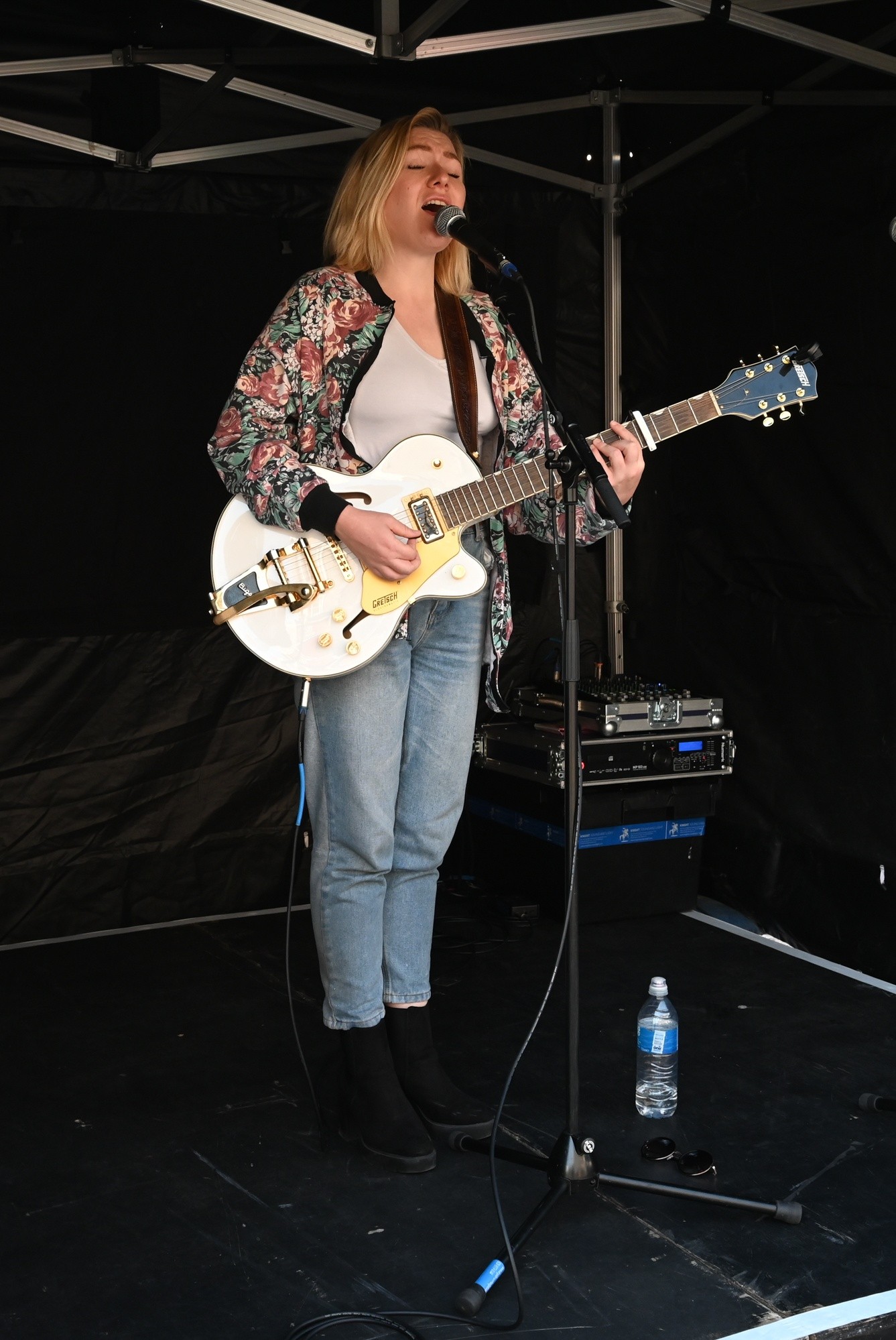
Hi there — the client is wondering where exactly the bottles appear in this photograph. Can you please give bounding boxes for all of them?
[635,977,679,1118]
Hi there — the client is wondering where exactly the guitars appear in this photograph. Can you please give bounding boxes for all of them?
[206,340,820,680]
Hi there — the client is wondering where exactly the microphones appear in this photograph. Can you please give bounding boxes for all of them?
[434,204,518,277]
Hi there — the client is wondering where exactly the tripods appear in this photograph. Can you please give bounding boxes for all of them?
[447,262,802,1320]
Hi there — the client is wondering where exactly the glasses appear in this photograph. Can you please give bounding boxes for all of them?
[640,1136,718,1178]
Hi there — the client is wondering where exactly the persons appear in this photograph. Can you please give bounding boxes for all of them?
[208,106,651,1170]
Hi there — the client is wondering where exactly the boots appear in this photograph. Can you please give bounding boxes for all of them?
[324,1015,438,1175]
[383,1001,495,1141]
